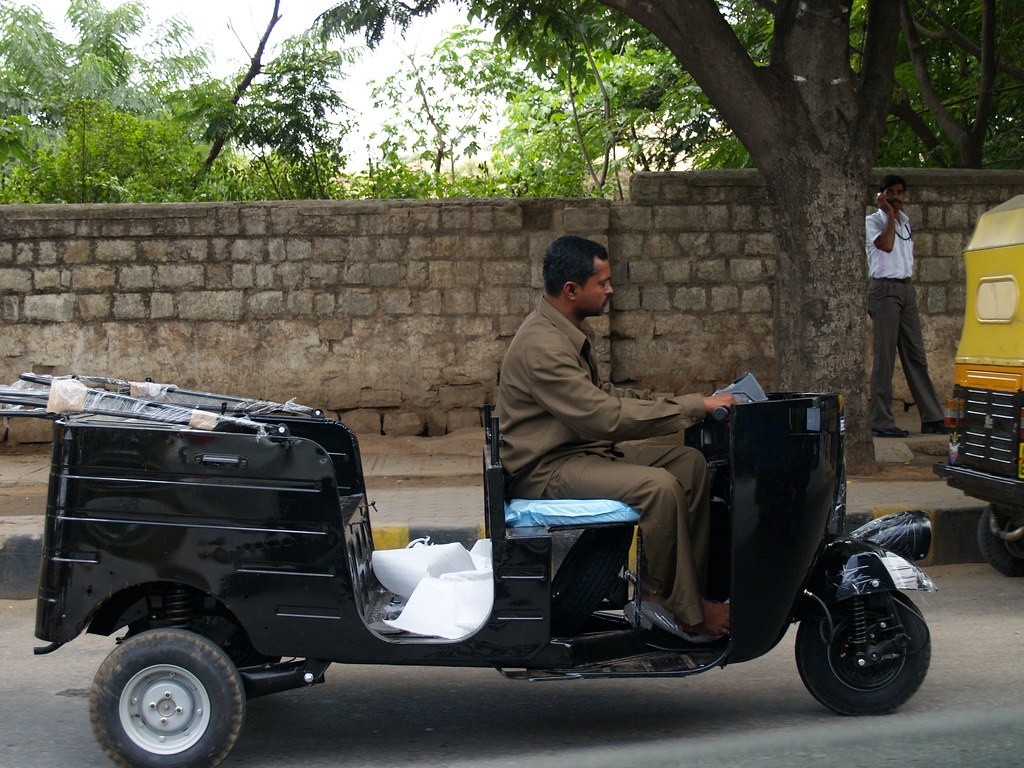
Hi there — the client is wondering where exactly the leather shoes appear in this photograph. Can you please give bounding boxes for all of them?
[869,426,909,438]
[921,420,963,435]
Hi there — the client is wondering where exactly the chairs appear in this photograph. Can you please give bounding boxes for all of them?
[474,402,646,632]
[211,412,368,542]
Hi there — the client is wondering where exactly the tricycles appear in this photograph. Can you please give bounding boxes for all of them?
[0,365,935,767]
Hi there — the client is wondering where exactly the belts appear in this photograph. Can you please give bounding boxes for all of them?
[873,277,911,284]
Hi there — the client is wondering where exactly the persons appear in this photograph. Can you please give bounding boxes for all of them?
[497,236,737,636]
[866,175,956,437]
